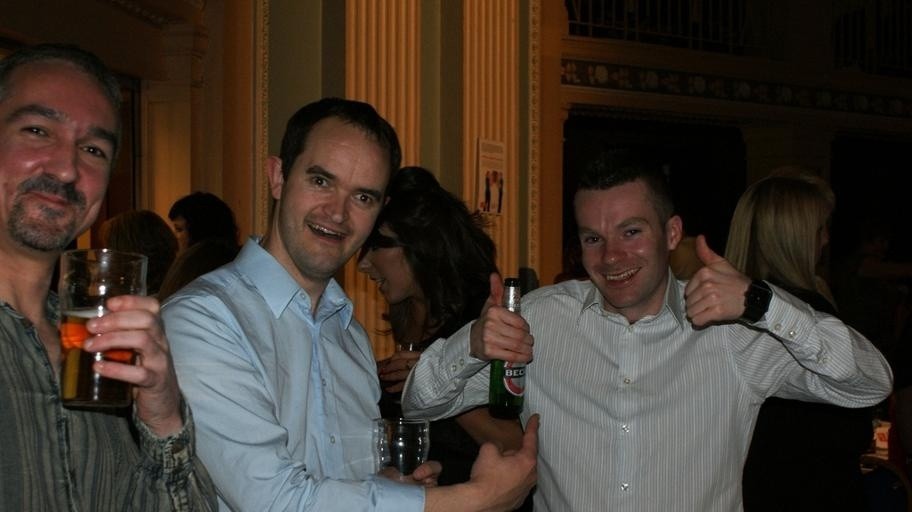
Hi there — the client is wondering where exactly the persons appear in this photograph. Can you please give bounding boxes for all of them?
[669,167,908,510]
[397,143,910,512]
[146,93,541,511]
[99,191,244,306]
[1,37,229,510]
[356,166,590,510]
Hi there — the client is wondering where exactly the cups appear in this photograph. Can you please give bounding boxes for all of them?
[57,248,148,410]
[373,418,428,485]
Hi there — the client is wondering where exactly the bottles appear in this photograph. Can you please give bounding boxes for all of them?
[486,278,527,419]
